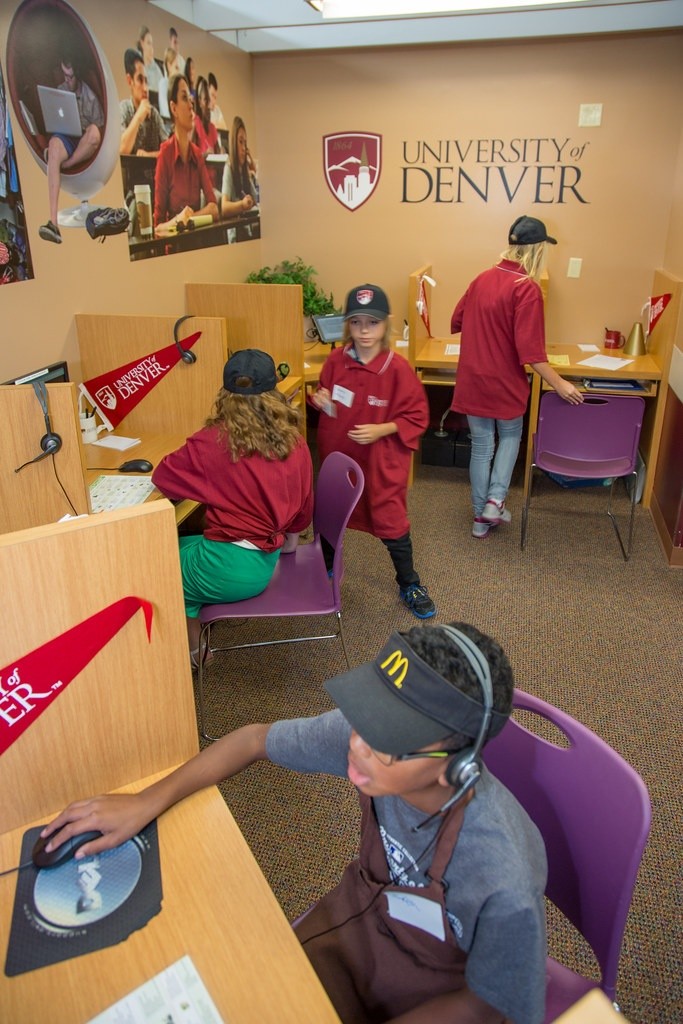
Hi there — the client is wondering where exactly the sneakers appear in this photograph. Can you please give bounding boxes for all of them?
[471,500,511,538]
[399,584,436,619]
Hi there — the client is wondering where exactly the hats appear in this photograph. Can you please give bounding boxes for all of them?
[323,629,511,754]
[342,283,391,321]
[223,348,277,394]
[508,215,558,246]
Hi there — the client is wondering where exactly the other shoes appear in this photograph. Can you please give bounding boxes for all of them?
[39,220,63,245]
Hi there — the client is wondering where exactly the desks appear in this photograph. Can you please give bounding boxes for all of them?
[119,116,260,261]
[0,266,683,1024]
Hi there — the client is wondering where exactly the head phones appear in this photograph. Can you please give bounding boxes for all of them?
[173,314,197,366]
[32,379,63,455]
[438,621,494,791]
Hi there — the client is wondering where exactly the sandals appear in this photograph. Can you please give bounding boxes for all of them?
[189,644,215,675]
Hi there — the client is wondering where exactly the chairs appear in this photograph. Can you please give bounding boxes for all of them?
[291,682,652,1024]
[198,449,366,743]
[5,0,123,226]
[519,390,645,562]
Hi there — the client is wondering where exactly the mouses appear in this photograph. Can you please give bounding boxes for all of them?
[31,820,103,869]
[119,459,154,473]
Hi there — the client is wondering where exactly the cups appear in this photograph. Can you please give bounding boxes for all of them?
[404,323,409,340]
[604,331,626,349]
[134,185,153,236]
[79,413,98,444]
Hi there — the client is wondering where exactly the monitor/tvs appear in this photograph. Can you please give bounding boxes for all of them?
[0,362,69,397]
[311,313,346,350]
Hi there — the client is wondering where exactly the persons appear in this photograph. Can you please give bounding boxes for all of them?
[308,285,436,619]
[451,215,586,539]
[178,348,314,675]
[42,619,547,1024]
[39,27,261,243]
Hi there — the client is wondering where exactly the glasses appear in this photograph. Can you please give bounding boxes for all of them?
[62,72,75,80]
[369,745,469,767]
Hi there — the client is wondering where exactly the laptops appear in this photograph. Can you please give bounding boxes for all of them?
[36,83,84,137]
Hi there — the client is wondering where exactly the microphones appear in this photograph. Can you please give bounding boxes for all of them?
[409,771,482,835]
[14,443,55,476]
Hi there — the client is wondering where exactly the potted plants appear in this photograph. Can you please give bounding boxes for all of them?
[244,255,343,345]
